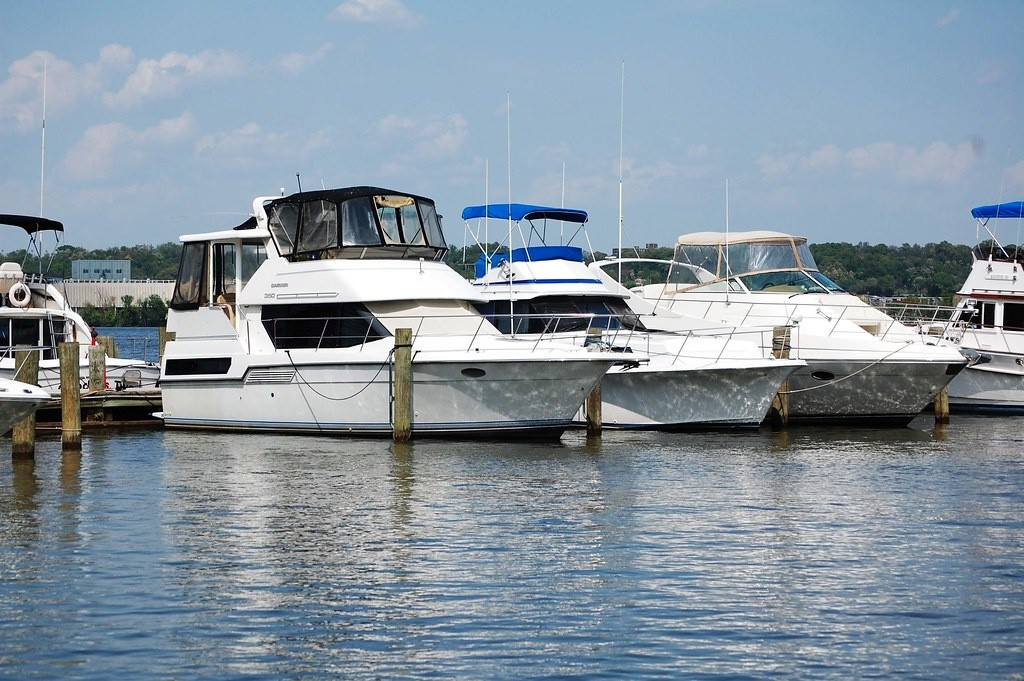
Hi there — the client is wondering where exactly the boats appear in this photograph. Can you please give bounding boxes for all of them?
[876,172,1024,409]
[0,63,162,425]
[162,60,966,437]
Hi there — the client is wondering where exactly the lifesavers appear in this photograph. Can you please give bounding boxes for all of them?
[9,282,32,307]
